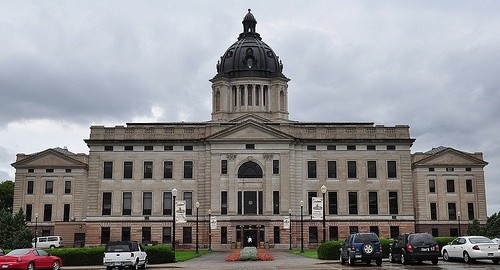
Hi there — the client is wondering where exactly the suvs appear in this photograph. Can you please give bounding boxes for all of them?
[339,231,382,266]
[388,232,439,265]
[102,240,148,270]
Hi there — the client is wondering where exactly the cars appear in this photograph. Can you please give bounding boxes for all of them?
[0,247,63,270]
[440,235,500,264]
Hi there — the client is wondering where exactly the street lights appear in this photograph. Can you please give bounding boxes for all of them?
[208,209,211,250]
[458,211,461,236]
[321,184,326,243]
[172,188,178,247]
[300,199,304,250]
[196,201,200,251]
[289,209,292,249]
[34,213,38,248]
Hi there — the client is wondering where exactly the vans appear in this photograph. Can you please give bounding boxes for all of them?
[31,236,64,250]
